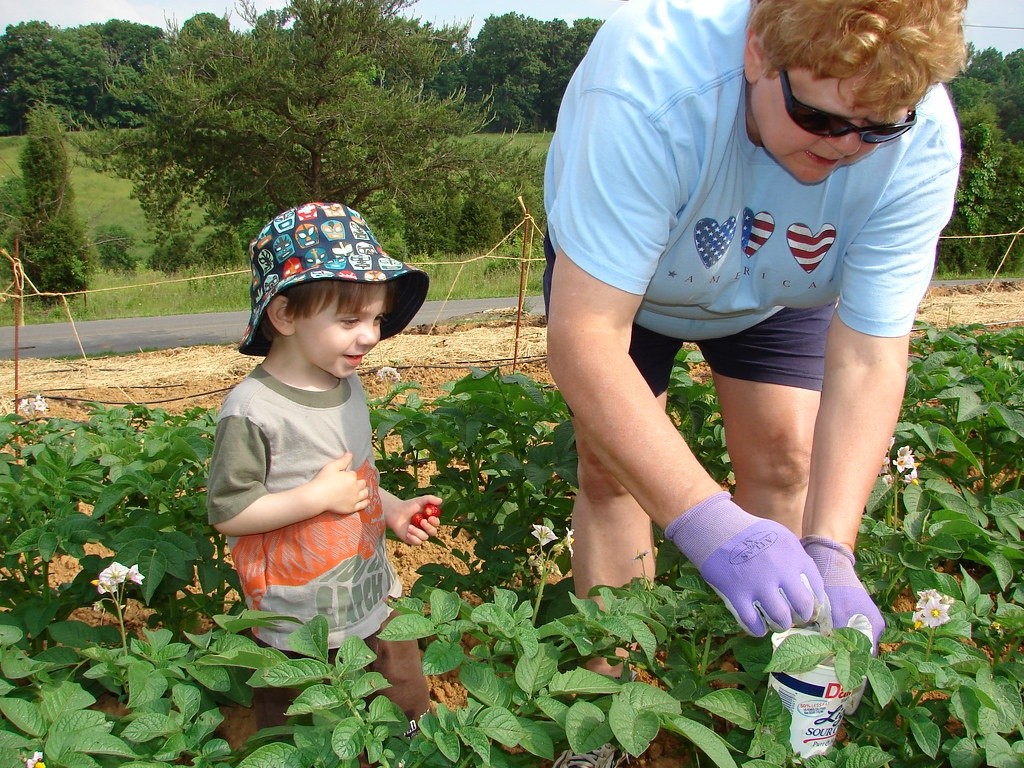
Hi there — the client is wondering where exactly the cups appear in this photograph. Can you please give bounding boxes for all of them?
[768,626,850,758]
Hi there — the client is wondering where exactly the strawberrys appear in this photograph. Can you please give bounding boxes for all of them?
[410,512,428,531]
[422,503,441,518]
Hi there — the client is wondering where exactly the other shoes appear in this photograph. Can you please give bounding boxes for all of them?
[551,741,630,768]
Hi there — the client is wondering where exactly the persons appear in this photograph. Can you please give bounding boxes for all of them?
[206,201,442,768]
[541,1,970,768]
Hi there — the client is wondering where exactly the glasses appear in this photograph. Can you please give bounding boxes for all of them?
[778,68,918,144]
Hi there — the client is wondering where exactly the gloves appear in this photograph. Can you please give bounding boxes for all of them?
[665,491,834,640]
[799,535,886,657]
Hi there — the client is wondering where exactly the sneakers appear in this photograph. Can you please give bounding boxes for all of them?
[237,201,430,356]
